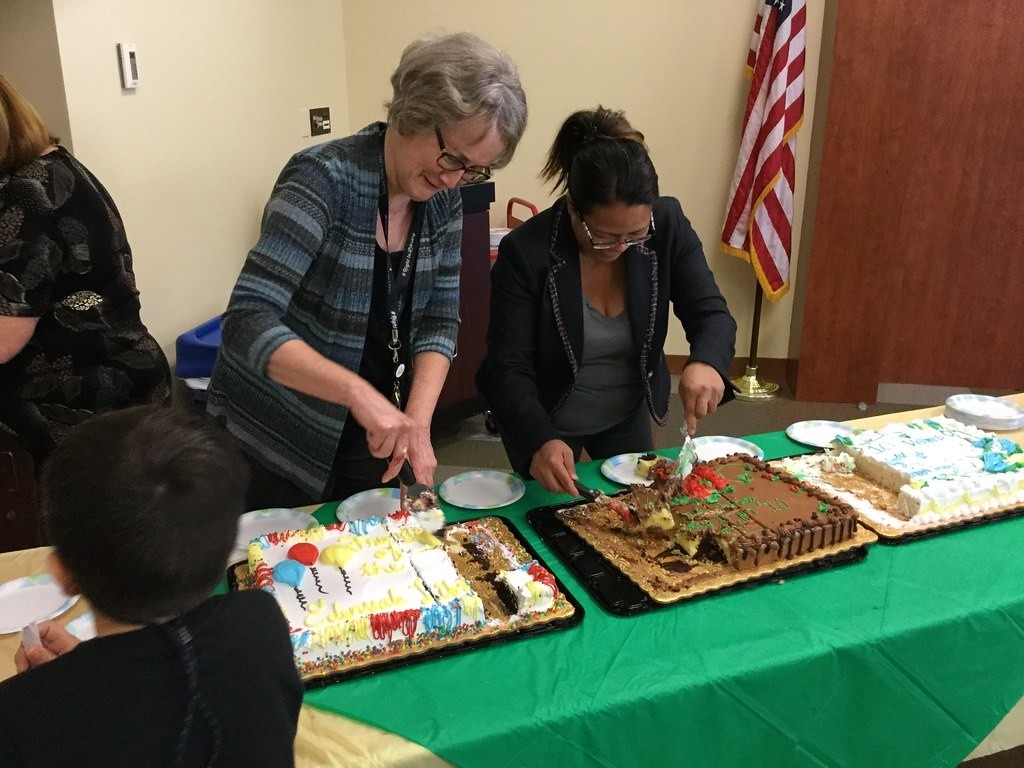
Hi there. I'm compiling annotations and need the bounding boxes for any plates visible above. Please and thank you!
[0,573,82,635]
[232,508,318,551]
[944,394,1023,431]
[437,470,526,510]
[336,487,402,522]
[600,453,676,485]
[691,435,764,461]
[785,419,859,448]
[63,610,97,641]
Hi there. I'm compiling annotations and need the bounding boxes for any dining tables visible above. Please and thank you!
[0,391,1023,768]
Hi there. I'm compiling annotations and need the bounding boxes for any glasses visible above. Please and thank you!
[574,206,656,250]
[435,126,491,185]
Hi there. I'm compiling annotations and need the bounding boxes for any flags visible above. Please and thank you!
[718,1,808,303]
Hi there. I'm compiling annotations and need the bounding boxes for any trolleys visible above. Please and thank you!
[489,197,537,265]
[427,179,503,447]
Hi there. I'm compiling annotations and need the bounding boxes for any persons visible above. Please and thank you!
[475,106,738,497]
[202,31,528,513]
[0,71,173,547]
[0,403,304,768]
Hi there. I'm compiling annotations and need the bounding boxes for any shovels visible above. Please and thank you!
[386,447,446,539]
[654,418,699,512]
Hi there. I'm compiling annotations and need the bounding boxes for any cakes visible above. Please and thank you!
[628,450,859,574]
[248,488,558,675]
[817,415,1023,523]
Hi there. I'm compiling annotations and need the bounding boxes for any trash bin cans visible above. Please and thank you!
[174,311,227,429]
